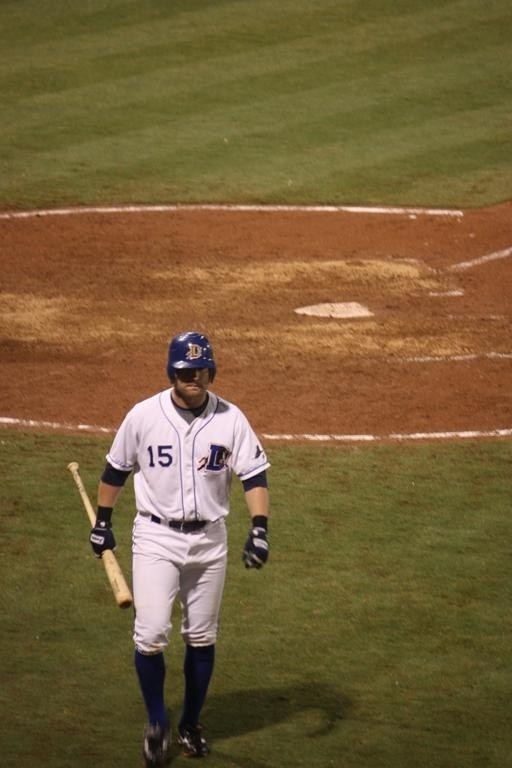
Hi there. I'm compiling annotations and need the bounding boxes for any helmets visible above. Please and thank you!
[167,332,216,377]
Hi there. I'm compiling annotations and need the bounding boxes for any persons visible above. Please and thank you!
[90,332,270,766]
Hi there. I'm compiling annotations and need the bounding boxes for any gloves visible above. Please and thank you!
[89,505,117,558]
[242,515,269,569]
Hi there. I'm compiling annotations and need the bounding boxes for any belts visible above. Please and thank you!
[151,515,207,531]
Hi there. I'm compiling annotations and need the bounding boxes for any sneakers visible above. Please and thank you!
[143,724,172,768]
[176,725,209,759]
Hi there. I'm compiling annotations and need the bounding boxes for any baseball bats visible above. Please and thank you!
[67,460,133,610]
